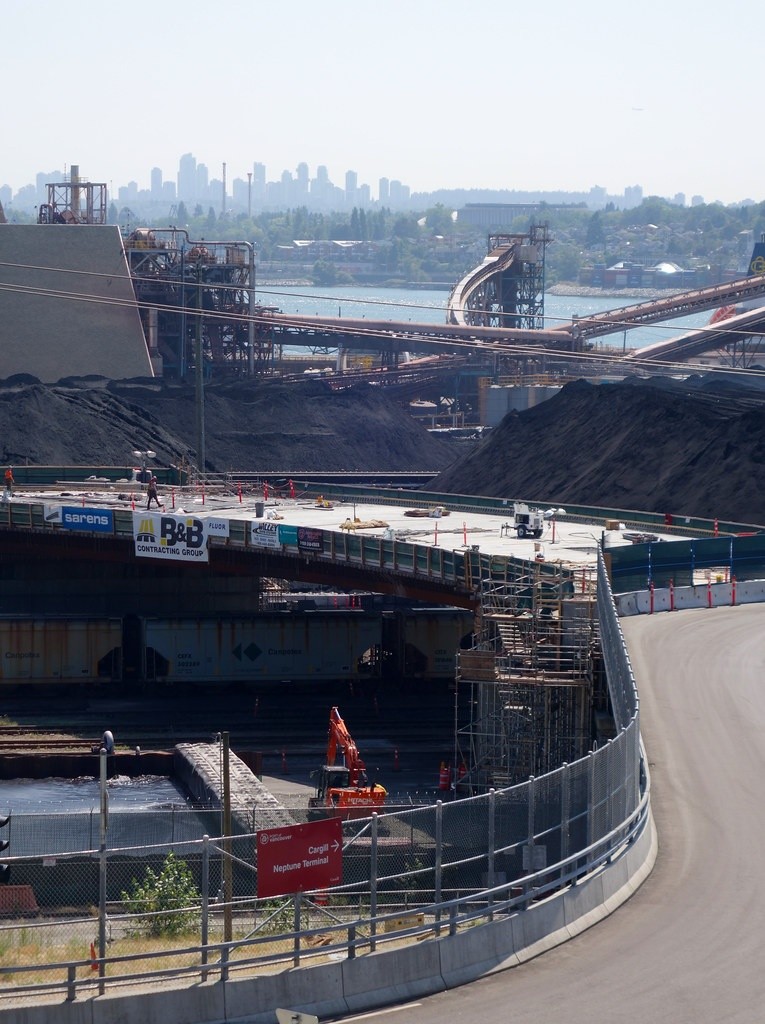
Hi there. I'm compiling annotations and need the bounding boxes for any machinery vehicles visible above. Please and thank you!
[513,502,545,538]
[307,707,390,838]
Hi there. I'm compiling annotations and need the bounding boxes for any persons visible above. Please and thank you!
[147,476,164,510]
[5,465,15,491]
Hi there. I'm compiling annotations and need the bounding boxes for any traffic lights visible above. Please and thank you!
[0,815,11,886]
